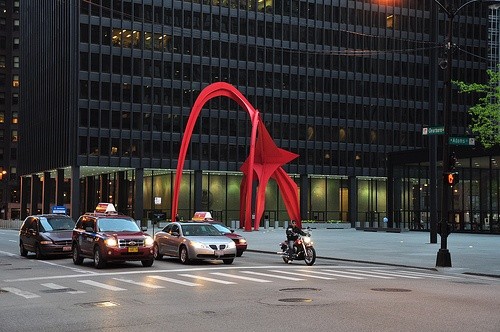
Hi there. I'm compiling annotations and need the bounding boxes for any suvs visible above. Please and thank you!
[72,212,157,270]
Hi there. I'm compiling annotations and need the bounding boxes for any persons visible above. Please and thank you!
[286,220,307,263]
[195,225,202,233]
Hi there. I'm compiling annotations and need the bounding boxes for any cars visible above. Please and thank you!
[154,221,236,264]
[189,212,214,223]
[209,222,247,256]
[19,214,75,259]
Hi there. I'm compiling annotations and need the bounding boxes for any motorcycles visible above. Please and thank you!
[278,226,316,266]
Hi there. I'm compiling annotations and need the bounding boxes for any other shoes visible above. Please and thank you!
[288,260,292,263]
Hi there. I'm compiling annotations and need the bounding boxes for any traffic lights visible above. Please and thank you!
[447,171,460,185]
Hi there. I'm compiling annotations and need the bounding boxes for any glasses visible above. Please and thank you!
[292,223,296,224]
